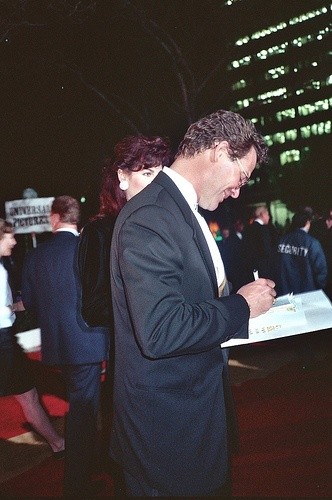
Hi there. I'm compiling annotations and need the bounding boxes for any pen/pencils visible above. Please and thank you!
[253,268,260,283]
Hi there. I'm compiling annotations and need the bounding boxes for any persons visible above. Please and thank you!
[208,201,332,296]
[108,111,277,497]
[78,134,171,497]
[274,212,327,295]
[0,218,66,462]
[20,197,108,497]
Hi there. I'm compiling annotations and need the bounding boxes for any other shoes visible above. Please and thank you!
[48,434,67,460]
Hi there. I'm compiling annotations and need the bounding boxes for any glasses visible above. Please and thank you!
[232,147,251,188]
